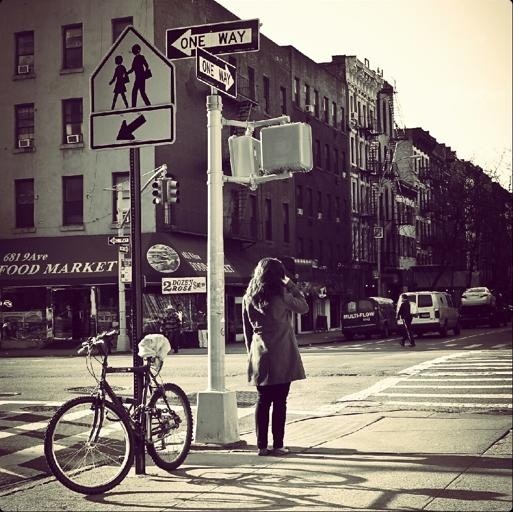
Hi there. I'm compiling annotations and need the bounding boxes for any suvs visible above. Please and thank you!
[394,291,462,339]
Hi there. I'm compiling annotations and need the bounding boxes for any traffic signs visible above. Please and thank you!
[165,17,261,61]
[194,45,239,100]
[117,244,129,253]
[107,235,130,246]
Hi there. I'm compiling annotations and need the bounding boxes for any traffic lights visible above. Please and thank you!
[163,177,180,206]
[151,177,165,206]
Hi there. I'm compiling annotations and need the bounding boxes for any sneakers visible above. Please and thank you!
[273,447,291,456]
[258,448,271,456]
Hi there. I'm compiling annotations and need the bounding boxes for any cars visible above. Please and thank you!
[459,286,497,310]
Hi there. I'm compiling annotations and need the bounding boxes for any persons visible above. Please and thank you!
[241,257,310,455]
[159,304,181,353]
[395,294,416,347]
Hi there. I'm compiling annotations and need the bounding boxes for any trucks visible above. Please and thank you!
[458,287,507,330]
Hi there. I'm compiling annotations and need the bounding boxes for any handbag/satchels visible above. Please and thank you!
[409,302,418,315]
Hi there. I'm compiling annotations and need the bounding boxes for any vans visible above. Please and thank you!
[339,295,398,341]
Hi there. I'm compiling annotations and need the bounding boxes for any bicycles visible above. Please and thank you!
[43,328,194,497]
